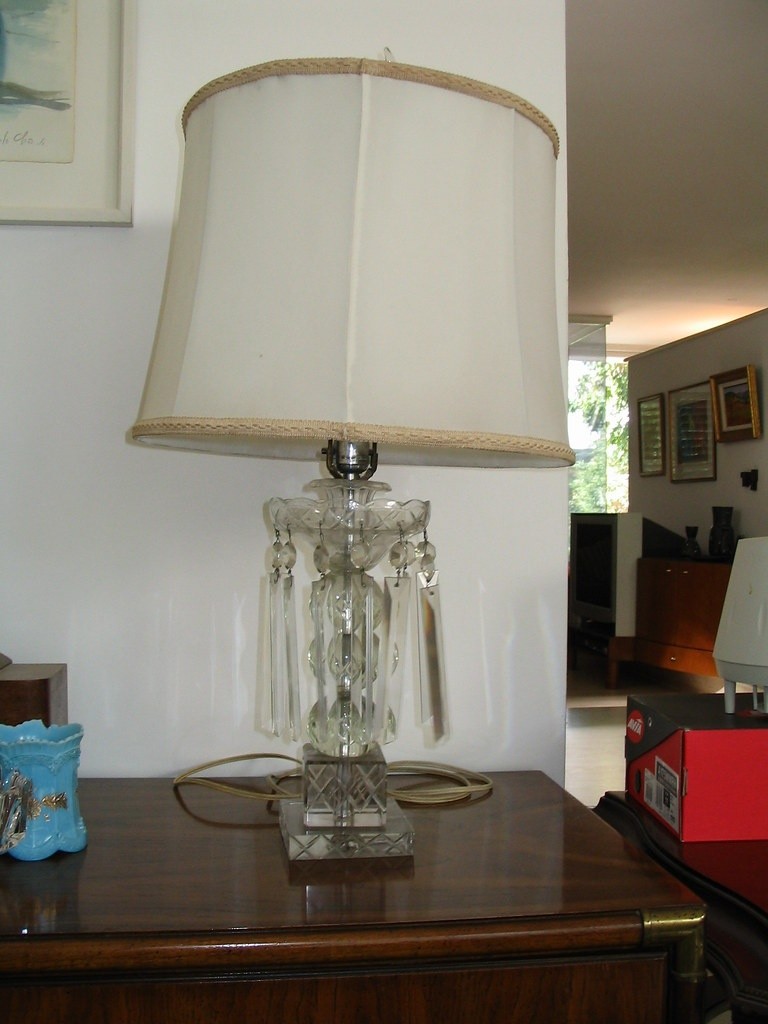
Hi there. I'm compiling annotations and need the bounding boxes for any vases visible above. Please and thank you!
[681,527,701,560]
[708,506,735,561]
[0,719,88,861]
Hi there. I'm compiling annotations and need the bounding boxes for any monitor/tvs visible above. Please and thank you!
[571,513,686,637]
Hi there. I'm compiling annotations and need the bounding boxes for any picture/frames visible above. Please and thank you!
[710,363,760,441]
[636,393,667,477]
[0,0,137,229]
[667,380,716,484]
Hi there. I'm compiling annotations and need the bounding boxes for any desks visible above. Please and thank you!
[592,790,768,1024]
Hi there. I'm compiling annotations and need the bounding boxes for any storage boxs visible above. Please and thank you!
[624,692,768,843]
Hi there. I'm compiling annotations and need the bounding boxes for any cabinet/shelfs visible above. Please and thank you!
[0,769,708,1024]
[636,558,733,677]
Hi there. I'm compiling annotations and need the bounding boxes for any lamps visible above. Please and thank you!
[128,56,577,862]
